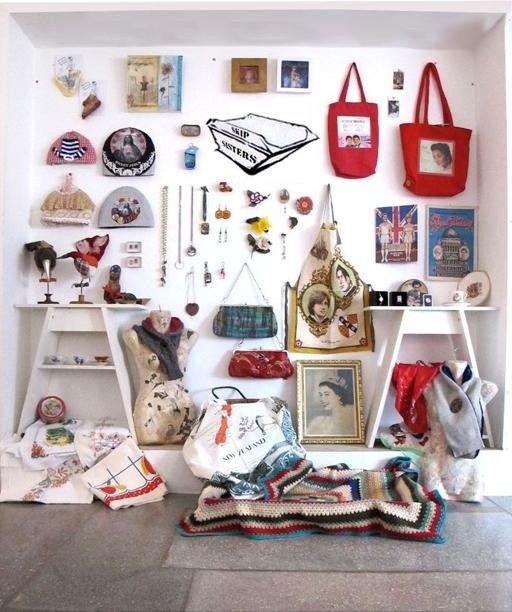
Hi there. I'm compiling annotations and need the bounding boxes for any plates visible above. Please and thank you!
[457,271,490,305]
[400,279,428,306]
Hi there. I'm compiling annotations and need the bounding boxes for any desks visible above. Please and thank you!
[15,303,146,444]
[362,306,498,449]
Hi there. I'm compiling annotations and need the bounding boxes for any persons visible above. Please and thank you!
[306,376,357,437]
[138,74,151,105]
[377,212,393,262]
[290,65,303,87]
[434,248,442,260]
[408,282,425,303]
[430,142,455,173]
[121,134,143,164]
[308,290,331,324]
[121,310,199,445]
[336,264,358,299]
[400,214,415,261]
[345,135,365,147]
[422,360,499,503]
[460,248,468,260]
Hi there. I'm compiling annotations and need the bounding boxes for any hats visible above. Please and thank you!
[98,186,154,228]
[47,131,97,164]
[41,174,96,225]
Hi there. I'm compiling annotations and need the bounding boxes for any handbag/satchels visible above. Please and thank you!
[399,122,472,197]
[328,102,378,179]
[182,398,298,482]
[229,351,295,379]
[213,306,279,337]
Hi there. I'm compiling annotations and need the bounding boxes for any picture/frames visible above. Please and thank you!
[297,359,363,444]
[277,57,313,95]
[425,205,479,282]
